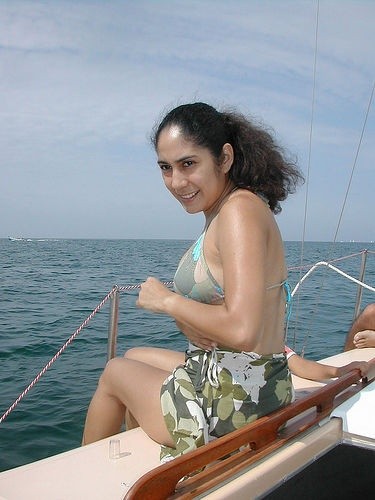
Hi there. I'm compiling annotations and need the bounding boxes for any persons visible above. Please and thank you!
[283,342,370,384]
[79,102,304,455]
[341,301,375,353]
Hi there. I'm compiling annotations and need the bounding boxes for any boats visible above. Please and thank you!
[8,236,32,242]
